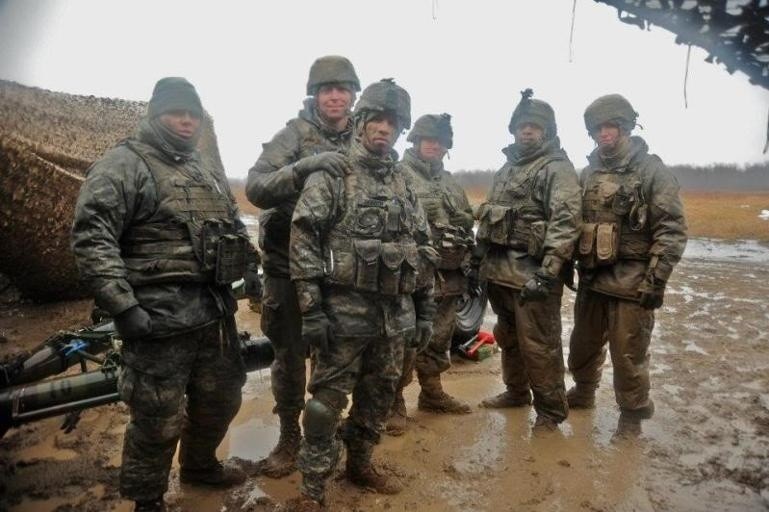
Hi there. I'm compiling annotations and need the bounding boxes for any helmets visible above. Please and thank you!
[406,114,452,150]
[352,79,411,130]
[509,99,556,139]
[307,55,360,95]
[583,95,637,132]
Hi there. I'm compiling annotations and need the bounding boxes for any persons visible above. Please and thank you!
[383,114,474,439]
[460,86,585,439]
[71,76,262,511]
[288,75,444,509]
[564,94,688,449]
[244,56,362,480]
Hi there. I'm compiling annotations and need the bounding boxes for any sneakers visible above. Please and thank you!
[613,410,641,440]
[479,389,532,407]
[531,414,557,440]
[563,386,594,409]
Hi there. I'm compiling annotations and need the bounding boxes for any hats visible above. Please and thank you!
[148,77,203,125]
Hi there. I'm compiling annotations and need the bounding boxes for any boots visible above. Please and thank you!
[180,449,245,486]
[266,406,302,476]
[418,371,471,414]
[385,384,408,432]
[346,440,404,494]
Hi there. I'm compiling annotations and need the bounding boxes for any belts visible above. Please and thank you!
[466,265,483,299]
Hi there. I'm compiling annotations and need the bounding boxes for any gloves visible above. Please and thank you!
[563,260,578,292]
[294,152,355,179]
[412,319,433,354]
[302,309,335,354]
[449,210,473,230]
[636,280,664,311]
[114,306,152,337]
[518,276,551,308]
[243,263,263,298]
